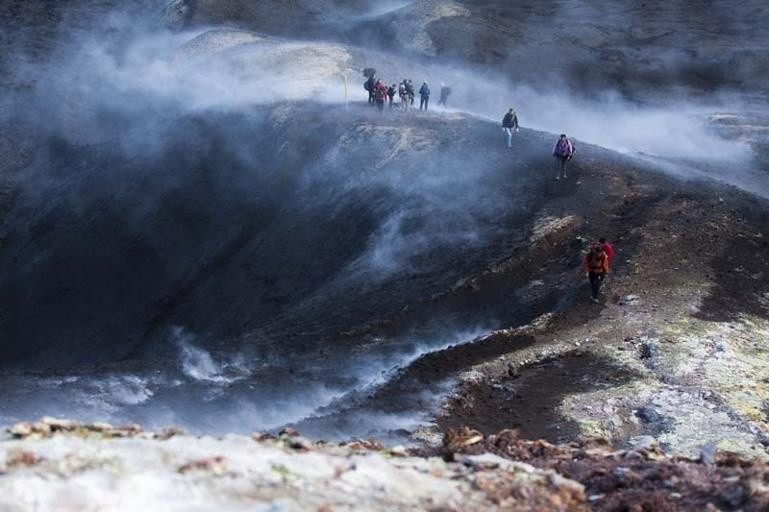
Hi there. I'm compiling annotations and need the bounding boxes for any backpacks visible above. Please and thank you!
[566,140,576,159]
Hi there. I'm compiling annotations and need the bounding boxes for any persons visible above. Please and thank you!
[419,81,430,112]
[583,238,613,304]
[437,82,450,108]
[366,74,414,112]
[502,108,519,150]
[552,134,573,180]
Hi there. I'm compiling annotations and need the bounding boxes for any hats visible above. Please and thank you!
[593,240,600,245]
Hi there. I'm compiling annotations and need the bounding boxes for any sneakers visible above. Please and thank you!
[590,295,600,304]
